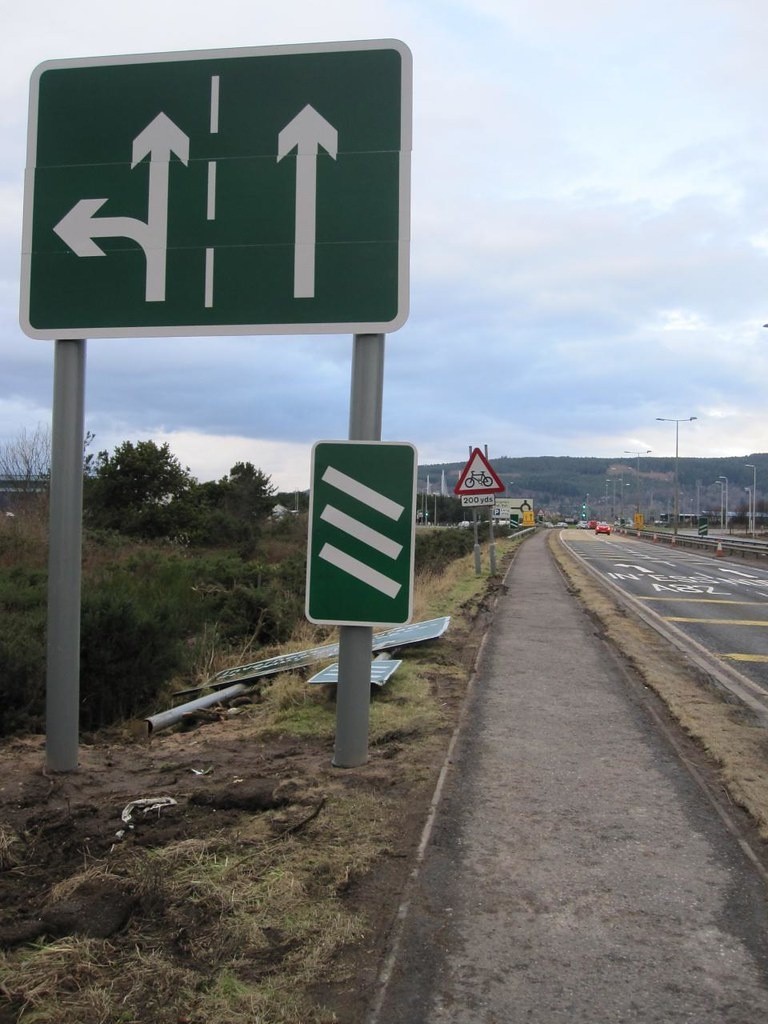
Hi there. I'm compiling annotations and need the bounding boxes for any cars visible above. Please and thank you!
[575,520,600,530]
[543,522,568,529]
[595,523,610,536]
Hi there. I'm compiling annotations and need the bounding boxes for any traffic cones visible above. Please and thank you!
[715,541,724,557]
[653,533,658,543]
[611,527,628,537]
[670,536,678,547]
[637,532,641,539]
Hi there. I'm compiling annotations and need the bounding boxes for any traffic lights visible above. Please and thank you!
[582,503,585,509]
[582,513,586,521]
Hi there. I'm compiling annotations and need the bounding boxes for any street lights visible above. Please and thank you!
[718,475,728,529]
[715,481,724,529]
[656,417,699,534]
[745,465,755,540]
[744,487,751,533]
[605,479,622,520]
[623,450,652,514]
